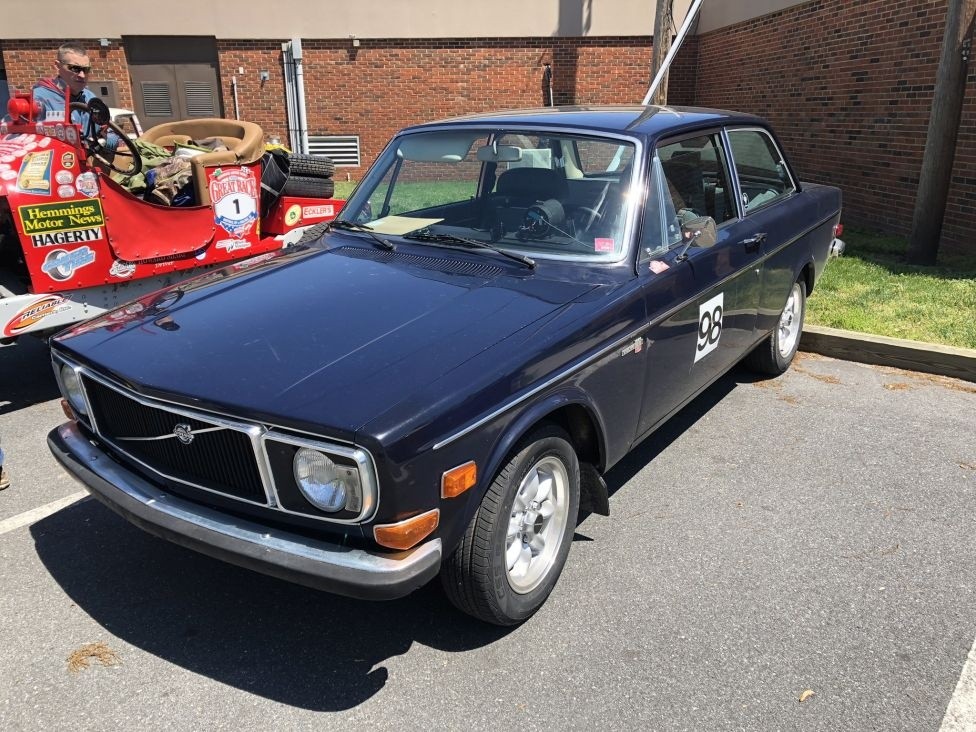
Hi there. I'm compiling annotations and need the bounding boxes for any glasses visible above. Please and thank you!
[58,60,92,75]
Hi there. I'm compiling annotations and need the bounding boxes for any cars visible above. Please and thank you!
[44,102,843,629]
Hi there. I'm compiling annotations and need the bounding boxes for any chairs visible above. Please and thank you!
[618,160,708,216]
[206,136,242,148]
[154,135,192,145]
[480,166,568,231]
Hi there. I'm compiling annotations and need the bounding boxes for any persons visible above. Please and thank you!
[610,162,707,254]
[31,43,119,175]
